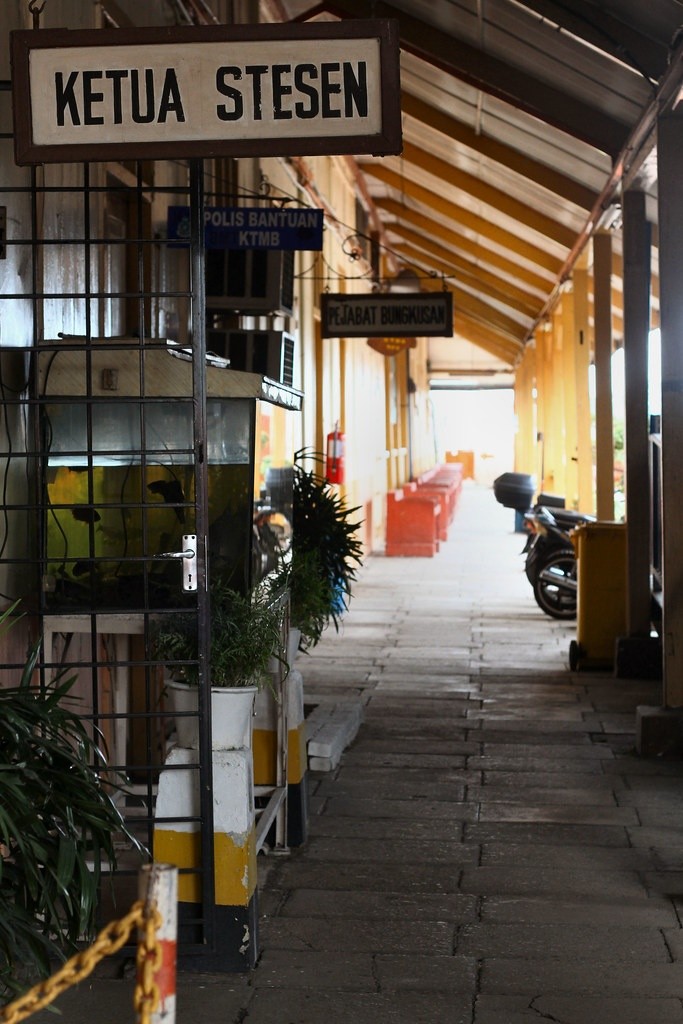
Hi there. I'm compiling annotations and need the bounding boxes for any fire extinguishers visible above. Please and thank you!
[327,420,345,485]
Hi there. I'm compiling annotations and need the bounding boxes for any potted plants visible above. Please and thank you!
[262,445,367,673]
[143,585,286,751]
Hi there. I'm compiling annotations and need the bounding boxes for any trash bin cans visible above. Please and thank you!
[567,522,628,671]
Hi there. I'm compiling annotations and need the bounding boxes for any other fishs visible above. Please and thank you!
[72,479,248,577]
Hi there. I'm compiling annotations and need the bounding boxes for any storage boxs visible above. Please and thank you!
[39,339,305,614]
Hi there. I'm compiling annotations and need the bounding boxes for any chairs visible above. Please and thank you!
[388,460,462,557]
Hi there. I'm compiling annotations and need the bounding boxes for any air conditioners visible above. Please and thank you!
[204,244,295,317]
[206,329,296,389]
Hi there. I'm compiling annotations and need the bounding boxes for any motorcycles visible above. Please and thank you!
[495,472,596,620]
